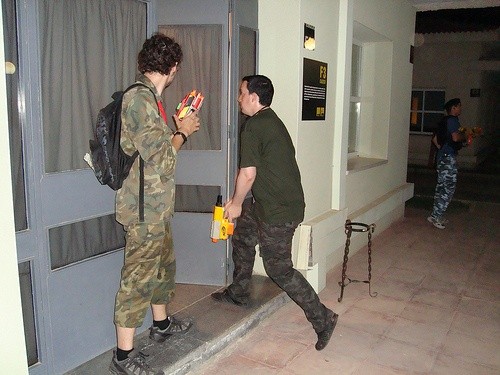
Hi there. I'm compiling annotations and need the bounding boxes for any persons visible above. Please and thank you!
[211,75,339,351]
[427,98,483,230]
[89,32,200,375]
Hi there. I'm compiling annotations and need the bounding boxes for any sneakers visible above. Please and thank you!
[148,315,192,344]
[427,216,446,229]
[108,348,165,375]
[441,218,448,224]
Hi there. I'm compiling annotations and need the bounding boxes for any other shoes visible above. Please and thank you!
[315,312,338,351]
[211,290,247,306]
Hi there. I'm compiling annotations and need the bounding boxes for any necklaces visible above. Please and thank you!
[252,105,269,117]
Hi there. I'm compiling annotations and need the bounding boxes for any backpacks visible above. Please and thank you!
[89,83,160,190]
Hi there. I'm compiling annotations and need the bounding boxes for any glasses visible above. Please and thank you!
[176,63,182,72]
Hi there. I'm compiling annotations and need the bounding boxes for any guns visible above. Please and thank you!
[176,88,205,133]
[210,195,235,243]
[454,126,483,144]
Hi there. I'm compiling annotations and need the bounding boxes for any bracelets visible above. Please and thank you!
[173,131,187,145]
